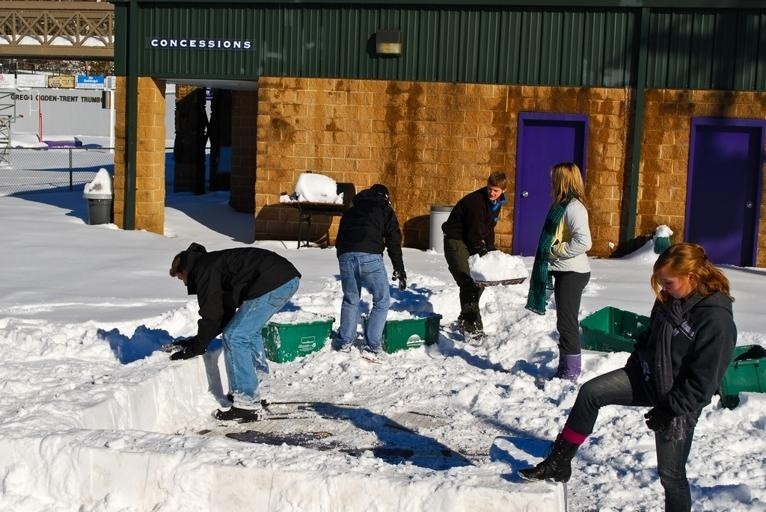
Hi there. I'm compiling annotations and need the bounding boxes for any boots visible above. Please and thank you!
[517,433,580,483]
[535,353,581,391]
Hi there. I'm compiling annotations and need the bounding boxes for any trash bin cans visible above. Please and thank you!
[428,205,458,254]
[83,183,114,225]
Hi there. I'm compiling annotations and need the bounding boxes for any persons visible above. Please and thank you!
[441,171,508,339]
[517,243,737,512]
[169,242,302,419]
[336,184,407,358]
[540,163,594,389]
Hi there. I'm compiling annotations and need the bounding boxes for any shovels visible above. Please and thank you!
[477,278,527,287]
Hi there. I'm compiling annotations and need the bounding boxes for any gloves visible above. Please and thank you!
[392,270,407,291]
[644,401,676,435]
[170,336,205,360]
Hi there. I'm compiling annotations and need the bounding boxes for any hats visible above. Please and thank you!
[370,184,391,205]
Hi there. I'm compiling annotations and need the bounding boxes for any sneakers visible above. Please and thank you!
[363,347,390,363]
[336,342,351,353]
[214,394,270,423]
